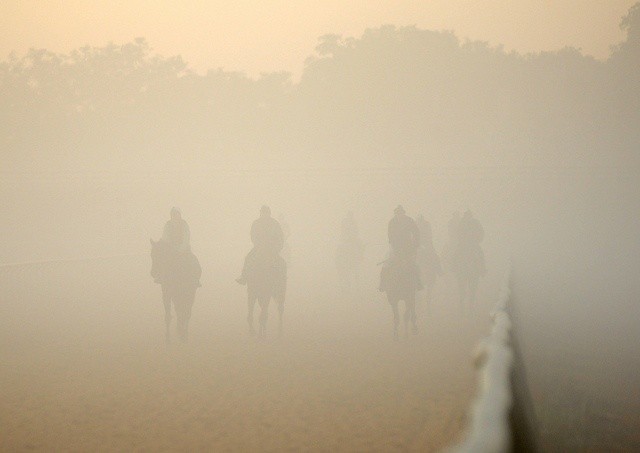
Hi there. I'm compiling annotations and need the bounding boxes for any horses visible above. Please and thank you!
[237,242,286,337]
[379,242,419,342]
[149,237,200,342]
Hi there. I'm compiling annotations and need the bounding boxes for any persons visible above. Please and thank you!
[236,207,284,284]
[162,208,202,287]
[379,205,421,294]
